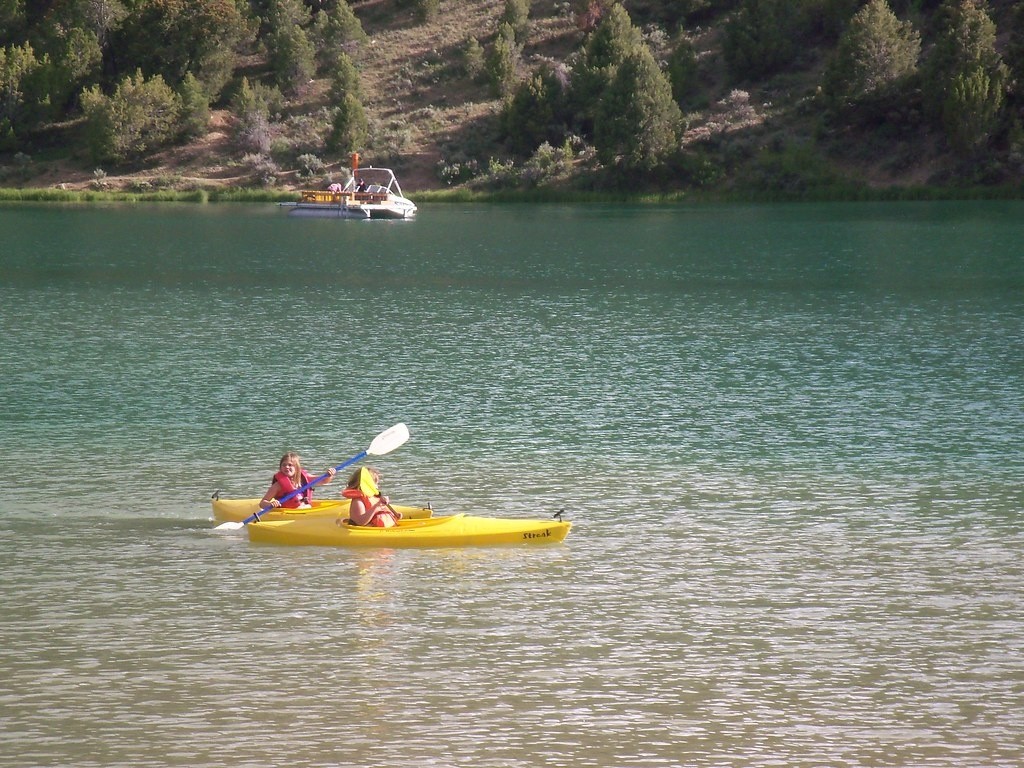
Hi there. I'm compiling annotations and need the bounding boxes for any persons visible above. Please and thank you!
[355,179,367,192]
[348,466,402,527]
[259,451,337,510]
[327,182,341,205]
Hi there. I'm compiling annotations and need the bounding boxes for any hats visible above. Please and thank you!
[359,179,363,182]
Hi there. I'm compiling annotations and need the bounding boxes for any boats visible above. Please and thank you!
[288,168,418,220]
[209,489,572,546]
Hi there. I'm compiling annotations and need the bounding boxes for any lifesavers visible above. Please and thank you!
[305,194,316,201]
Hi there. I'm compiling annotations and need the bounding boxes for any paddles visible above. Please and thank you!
[359,465,400,518]
[214,422,411,531]
